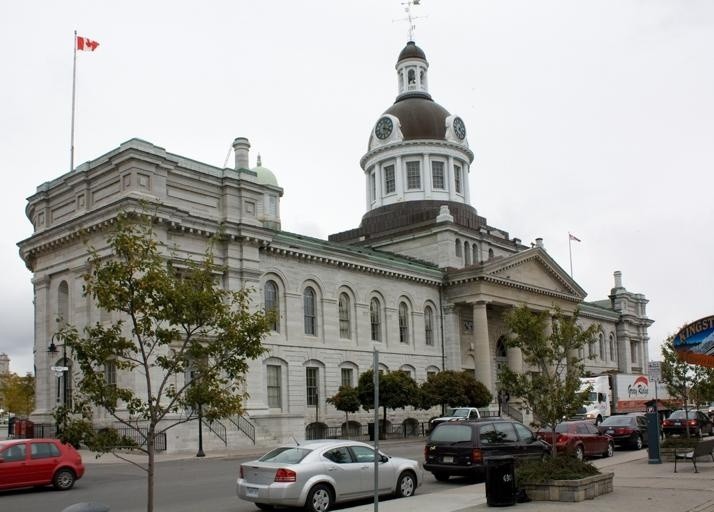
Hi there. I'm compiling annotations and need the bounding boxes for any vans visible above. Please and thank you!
[421,416,554,480]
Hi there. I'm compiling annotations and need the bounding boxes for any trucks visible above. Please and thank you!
[561,371,684,422]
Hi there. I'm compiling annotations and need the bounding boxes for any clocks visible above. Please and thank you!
[375,116,394,140]
[452,116,467,141]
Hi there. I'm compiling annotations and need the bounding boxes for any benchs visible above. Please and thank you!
[673,439,714,476]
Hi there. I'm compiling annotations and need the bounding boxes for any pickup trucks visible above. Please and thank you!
[430,406,481,430]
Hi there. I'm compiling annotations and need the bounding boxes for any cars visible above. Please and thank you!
[661,409,714,439]
[531,420,615,463]
[235,435,425,512]
[596,415,664,450]
[0,437,84,492]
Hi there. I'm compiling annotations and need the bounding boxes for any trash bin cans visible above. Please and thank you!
[8,417,33,439]
[367,422,383,441]
[483,454,517,507]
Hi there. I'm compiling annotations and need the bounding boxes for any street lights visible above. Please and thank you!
[46,332,68,428]
[191,370,206,458]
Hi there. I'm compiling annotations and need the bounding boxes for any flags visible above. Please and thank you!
[76,36,99,52]
[569,234,580,242]
